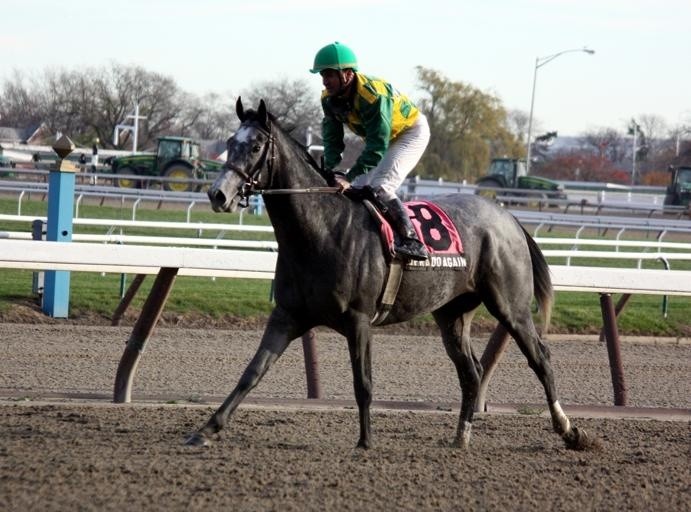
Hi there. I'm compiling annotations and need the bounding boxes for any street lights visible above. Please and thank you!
[524,44,598,179]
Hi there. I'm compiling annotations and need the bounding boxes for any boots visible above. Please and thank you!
[382,197,428,260]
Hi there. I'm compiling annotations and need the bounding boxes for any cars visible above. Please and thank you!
[0,146,14,179]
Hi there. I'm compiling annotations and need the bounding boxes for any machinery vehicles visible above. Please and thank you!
[112,137,230,194]
[474,157,569,211]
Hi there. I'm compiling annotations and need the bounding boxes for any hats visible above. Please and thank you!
[308,39,358,75]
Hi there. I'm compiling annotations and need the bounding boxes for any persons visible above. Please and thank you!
[308,41,433,261]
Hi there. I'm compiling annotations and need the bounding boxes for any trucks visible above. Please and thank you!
[664,164,691,218]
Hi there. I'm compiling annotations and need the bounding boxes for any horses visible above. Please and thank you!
[186,95,589,452]
